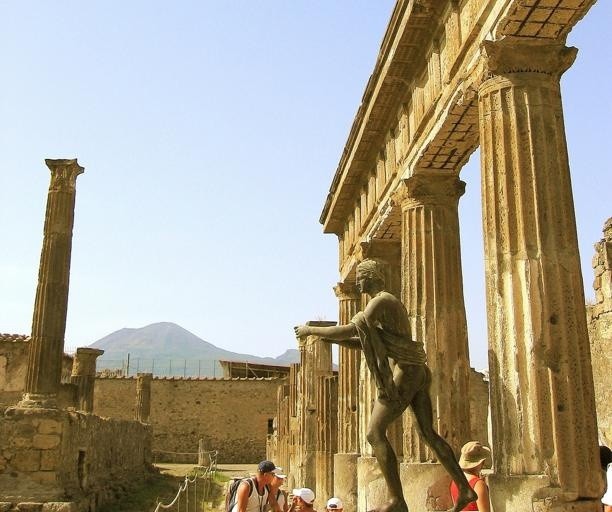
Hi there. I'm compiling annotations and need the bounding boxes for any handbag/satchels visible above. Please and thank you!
[226,478,253,512]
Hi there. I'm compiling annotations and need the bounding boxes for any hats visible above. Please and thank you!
[292,488,315,505]
[257,461,282,474]
[327,498,343,510]
[272,467,287,479]
[459,441,491,470]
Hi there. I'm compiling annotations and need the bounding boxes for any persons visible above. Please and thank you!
[449,441,490,512]
[294,260,478,512]
[601,446,612,512]
[232,461,343,512]
